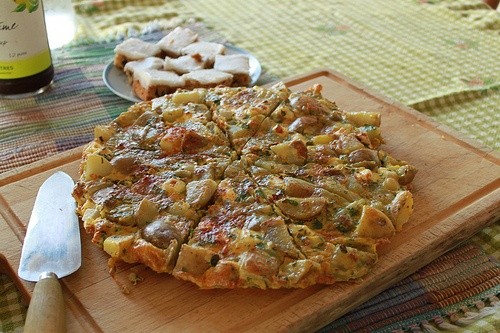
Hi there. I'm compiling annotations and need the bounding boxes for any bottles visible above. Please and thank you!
[0,0,53,98]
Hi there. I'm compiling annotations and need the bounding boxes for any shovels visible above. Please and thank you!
[17,170,82,333]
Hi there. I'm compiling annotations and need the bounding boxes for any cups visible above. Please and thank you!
[43,1,77,49]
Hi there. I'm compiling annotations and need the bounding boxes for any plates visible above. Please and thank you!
[103,38,262,104]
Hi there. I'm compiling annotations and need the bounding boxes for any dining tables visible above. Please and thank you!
[0,0,499,333]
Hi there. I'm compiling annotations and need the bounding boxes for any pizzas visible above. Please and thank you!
[70,84,417,291]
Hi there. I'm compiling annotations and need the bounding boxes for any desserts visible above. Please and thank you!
[112,25,252,101]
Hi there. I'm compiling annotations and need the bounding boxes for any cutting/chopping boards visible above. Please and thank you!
[3,67,499,332]
